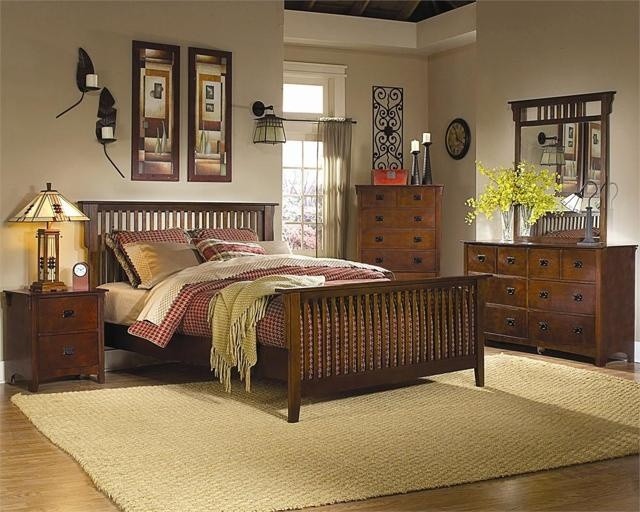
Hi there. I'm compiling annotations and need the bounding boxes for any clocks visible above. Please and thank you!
[445,118,471,161]
[72,262,90,292]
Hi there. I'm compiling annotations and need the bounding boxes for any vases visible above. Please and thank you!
[500,204,515,243]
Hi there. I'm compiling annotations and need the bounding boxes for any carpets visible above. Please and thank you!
[10,352,639,512]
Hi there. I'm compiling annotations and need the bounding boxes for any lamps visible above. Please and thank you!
[560,181,603,246]
[538,132,566,166]
[253,101,286,146]
[596,201,601,209]
[7,182,91,294]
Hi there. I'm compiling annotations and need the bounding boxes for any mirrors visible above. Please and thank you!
[507,90,618,242]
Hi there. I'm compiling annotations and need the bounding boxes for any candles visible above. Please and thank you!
[411,139,419,152]
[423,132,431,144]
[86,74,98,88]
[101,126,114,139]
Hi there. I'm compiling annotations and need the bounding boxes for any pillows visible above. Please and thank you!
[105,226,267,290]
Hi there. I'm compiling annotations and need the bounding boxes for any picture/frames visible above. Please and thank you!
[557,120,584,200]
[187,47,232,182]
[131,40,180,181]
[582,123,602,199]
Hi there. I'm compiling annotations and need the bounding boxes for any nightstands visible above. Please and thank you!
[3,289,110,393]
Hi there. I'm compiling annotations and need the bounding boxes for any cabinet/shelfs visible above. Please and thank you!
[463,241,638,368]
[354,183,445,279]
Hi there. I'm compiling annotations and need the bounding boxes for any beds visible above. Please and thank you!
[76,200,493,423]
